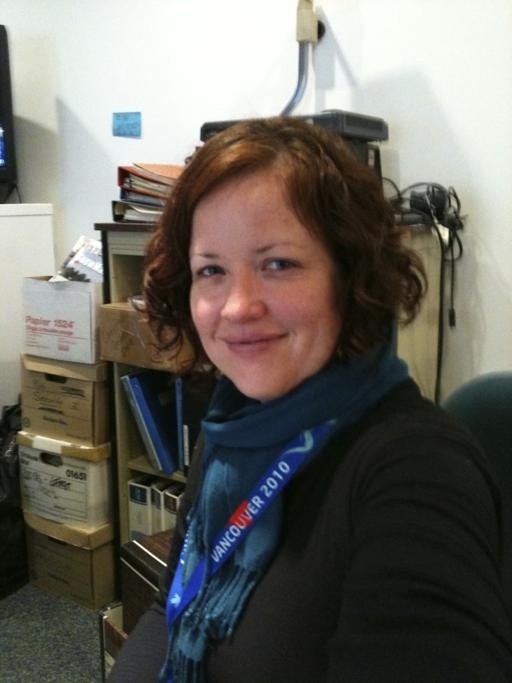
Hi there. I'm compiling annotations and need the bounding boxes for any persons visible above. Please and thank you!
[110,113,511,683]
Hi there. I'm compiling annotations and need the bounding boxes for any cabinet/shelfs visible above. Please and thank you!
[92,221,443,603]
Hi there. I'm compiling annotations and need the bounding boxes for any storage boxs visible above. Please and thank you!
[17,428,115,551]
[15,274,103,367]
[98,298,196,378]
[23,519,117,610]
[16,352,111,447]
